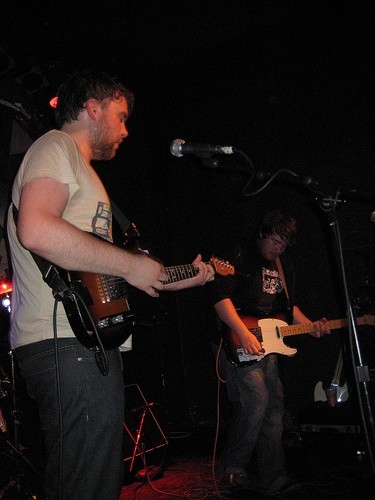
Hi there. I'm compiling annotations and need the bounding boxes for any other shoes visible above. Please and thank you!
[226,470,249,491]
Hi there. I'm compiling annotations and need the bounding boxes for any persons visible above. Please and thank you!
[210,212,333,491]
[4,70,215,500]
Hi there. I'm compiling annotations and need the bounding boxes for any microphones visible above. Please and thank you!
[130,402,158,414]
[170,137,237,160]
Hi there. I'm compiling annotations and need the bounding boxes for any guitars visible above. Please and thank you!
[313,347,350,404]
[230,301,375,365]
[63,229,236,349]
